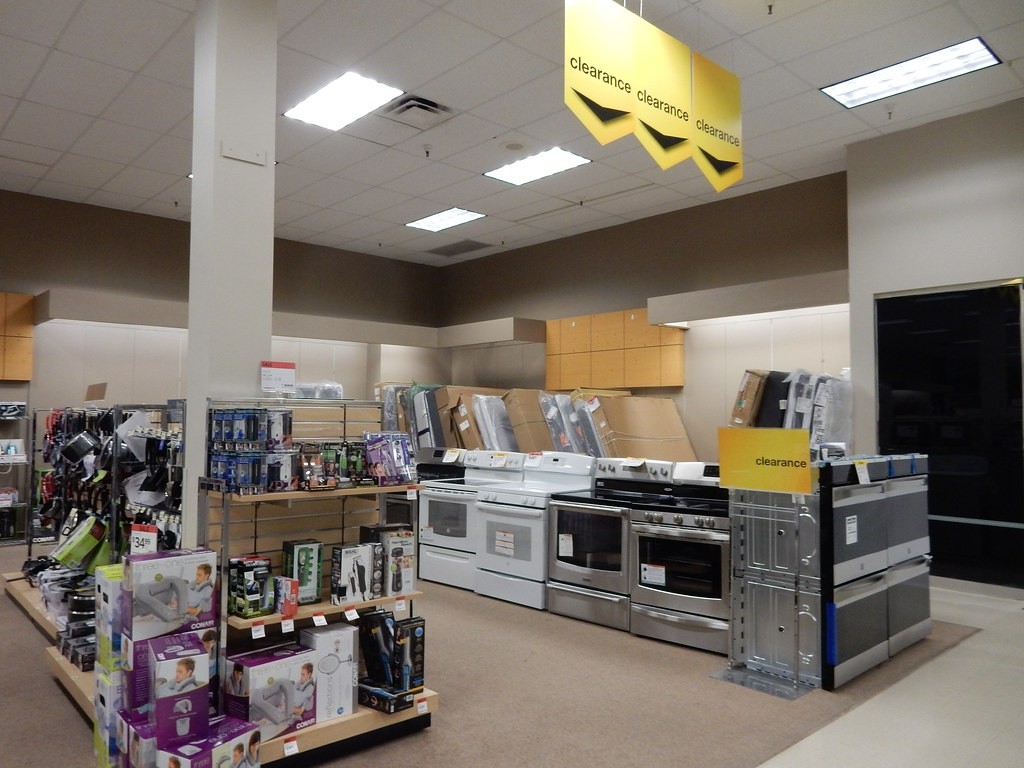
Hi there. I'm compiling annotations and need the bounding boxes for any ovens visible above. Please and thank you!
[737,454,933,690]
[386,446,469,533]
[544,456,674,635]
[416,449,527,591]
[470,449,596,610]
[629,461,731,658]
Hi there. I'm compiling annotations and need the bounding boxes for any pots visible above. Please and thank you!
[23,406,146,627]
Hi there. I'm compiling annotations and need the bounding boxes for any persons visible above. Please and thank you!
[127,731,142,766]
[214,744,247,768]
[154,659,196,697]
[237,730,262,768]
[116,638,134,675]
[168,756,181,768]
[249,660,317,728]
[135,564,215,625]
[199,629,217,676]
[226,662,247,700]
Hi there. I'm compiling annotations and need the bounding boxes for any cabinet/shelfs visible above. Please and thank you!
[3,399,439,768]
